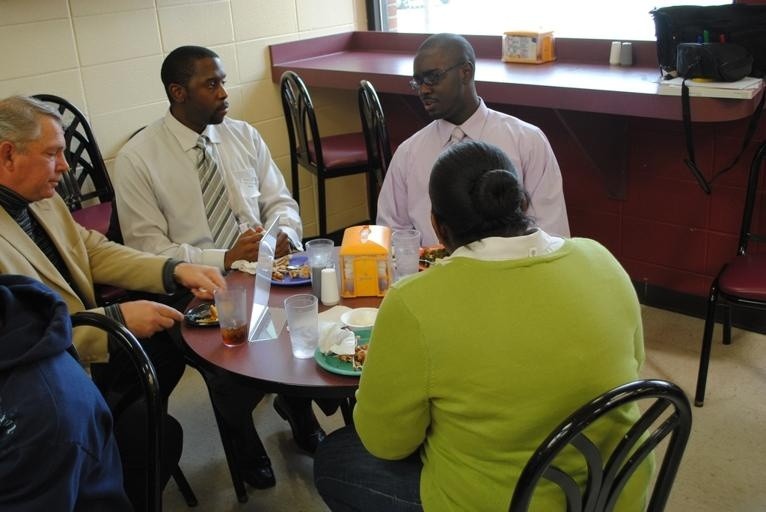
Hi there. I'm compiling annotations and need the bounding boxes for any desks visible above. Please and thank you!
[268,31,765,122]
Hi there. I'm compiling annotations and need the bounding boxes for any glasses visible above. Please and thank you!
[408,60,466,91]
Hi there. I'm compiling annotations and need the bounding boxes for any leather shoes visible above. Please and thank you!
[273,394,329,458]
[229,426,277,490]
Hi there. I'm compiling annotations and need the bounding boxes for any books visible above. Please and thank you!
[656,75,763,99]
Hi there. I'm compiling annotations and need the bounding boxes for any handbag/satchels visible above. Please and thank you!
[652,4,766,82]
[675,41,754,82]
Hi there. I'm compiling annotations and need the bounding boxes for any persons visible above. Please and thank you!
[114,45,328,489]
[314,140,656,511]
[376,32,571,247]
[1,275,136,511]
[0,94,227,432]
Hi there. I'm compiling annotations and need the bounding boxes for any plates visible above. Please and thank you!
[185,303,219,327]
[314,330,372,376]
[258,255,311,285]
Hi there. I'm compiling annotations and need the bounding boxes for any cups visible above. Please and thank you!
[392,229,421,282]
[305,239,334,297]
[213,286,248,348]
[341,308,379,331]
[284,294,319,359]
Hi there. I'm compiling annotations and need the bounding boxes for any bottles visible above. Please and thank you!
[320,269,340,306]
[610,42,621,64]
[622,43,632,64]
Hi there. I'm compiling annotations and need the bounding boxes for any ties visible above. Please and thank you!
[195,136,243,249]
[450,127,466,146]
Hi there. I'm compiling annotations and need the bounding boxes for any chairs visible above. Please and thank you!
[694,143,766,407]
[357,80,394,222]
[62,312,161,511]
[509,378,692,512]
[279,71,406,238]
[25,94,124,246]
